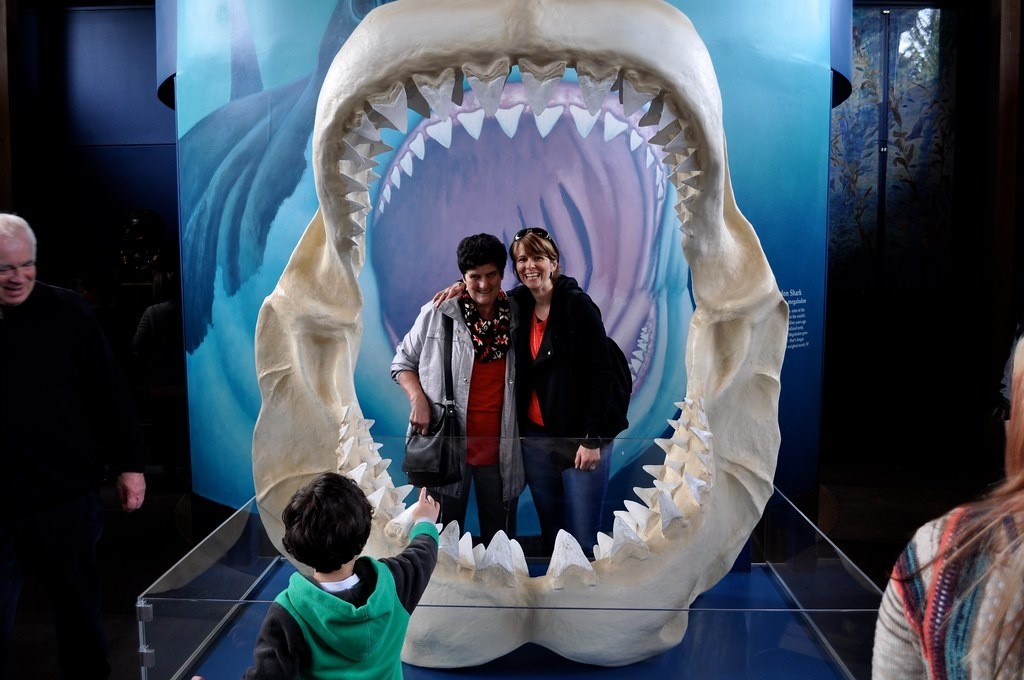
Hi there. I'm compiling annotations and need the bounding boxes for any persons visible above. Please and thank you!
[0,212,147,680]
[193,472,439,680]
[870,332,1024,680]
[391,232,522,552]
[434,228,612,562]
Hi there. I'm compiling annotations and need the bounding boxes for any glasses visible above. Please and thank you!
[514,228,549,241]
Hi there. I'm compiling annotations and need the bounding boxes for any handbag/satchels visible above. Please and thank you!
[402,313,462,488]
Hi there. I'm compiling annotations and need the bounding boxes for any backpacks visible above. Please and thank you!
[582,337,633,449]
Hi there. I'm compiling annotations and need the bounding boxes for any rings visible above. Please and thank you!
[442,291,448,296]
[590,466,595,469]
